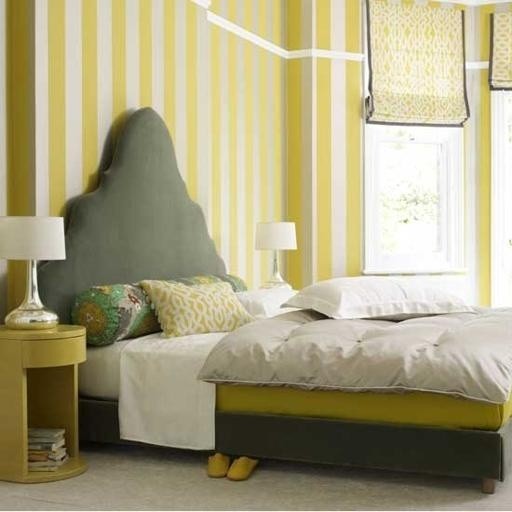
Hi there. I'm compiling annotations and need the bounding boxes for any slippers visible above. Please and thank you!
[208,453,230,477]
[227,456,258,480]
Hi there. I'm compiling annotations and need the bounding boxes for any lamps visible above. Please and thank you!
[255,221,297,289]
[1,215,67,329]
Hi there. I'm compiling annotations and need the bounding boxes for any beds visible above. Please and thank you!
[36,105,511,495]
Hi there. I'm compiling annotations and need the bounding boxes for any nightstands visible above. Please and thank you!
[1,324,89,483]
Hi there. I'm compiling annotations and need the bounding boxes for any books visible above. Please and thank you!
[28,428,72,472]
